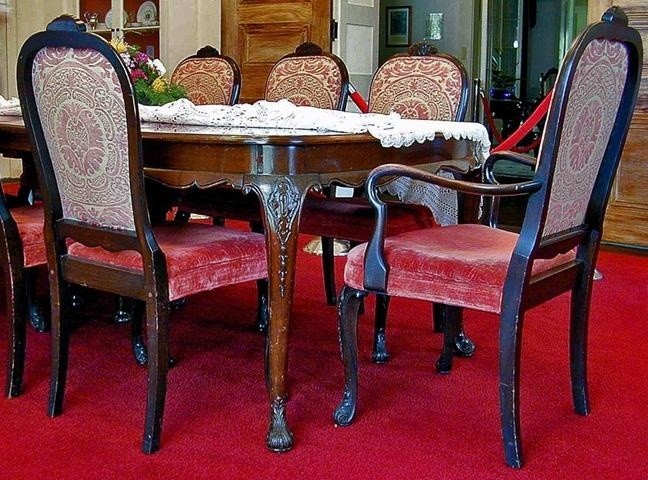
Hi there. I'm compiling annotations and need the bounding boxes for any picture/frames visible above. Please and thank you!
[383,6,413,47]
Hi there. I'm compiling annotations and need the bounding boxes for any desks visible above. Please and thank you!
[485,90,524,138]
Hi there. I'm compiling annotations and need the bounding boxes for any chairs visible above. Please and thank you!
[15,14,271,457]
[333,6,643,469]
[1,185,151,401]
[348,43,476,364]
[539,67,558,100]
[175,42,345,326]
[161,44,240,226]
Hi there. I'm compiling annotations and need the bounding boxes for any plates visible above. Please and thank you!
[104,8,128,28]
[136,1,156,26]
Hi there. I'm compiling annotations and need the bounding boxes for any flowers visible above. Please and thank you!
[106,32,187,108]
